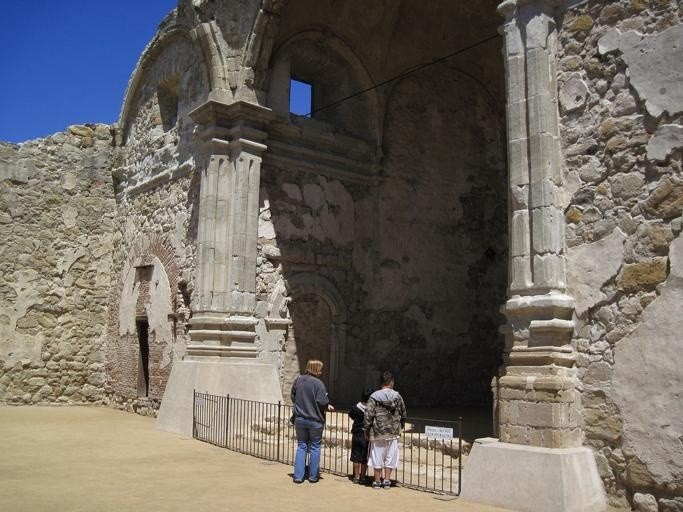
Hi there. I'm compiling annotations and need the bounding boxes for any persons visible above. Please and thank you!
[363,371,407,490]
[348,386,374,484]
[290,359,335,484]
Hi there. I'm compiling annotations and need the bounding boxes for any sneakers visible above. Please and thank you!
[294,475,318,483]
[372,481,382,488]
[353,479,368,484]
[383,480,391,488]
